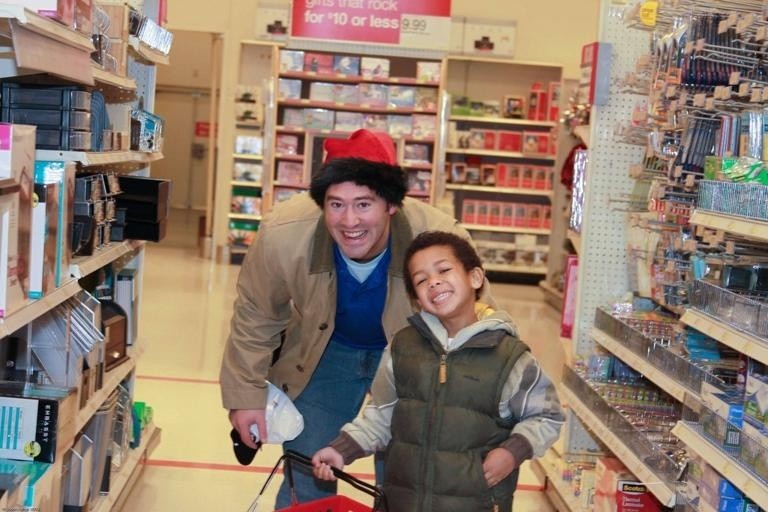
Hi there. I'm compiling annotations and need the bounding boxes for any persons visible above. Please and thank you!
[220,128,501,510]
[311,231,566,512]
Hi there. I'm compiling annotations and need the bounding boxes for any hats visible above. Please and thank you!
[310,130,409,209]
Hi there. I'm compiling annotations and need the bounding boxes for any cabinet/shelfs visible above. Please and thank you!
[2,0,161,511]
[223,39,566,278]
[529,0,768,512]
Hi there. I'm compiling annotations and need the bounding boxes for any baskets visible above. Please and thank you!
[247,450,390,512]
